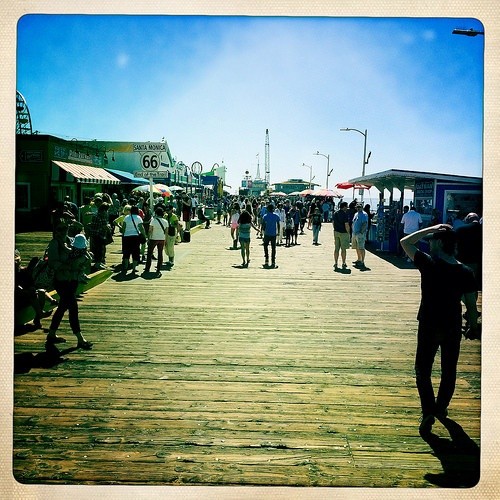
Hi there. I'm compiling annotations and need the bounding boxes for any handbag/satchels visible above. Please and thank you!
[167,225,176,237]
[99,225,113,246]
[138,232,146,245]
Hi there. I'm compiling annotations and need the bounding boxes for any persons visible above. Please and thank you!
[398,224,478,418]
[454,211,482,326]
[16,225,92,354]
[61,189,421,273]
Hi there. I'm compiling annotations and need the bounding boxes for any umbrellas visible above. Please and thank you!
[135,183,184,197]
[337,181,372,200]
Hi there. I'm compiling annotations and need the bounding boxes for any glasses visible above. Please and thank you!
[55,226,68,232]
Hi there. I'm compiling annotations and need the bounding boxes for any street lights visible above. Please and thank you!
[314,150,332,190]
[339,127,371,203]
[301,163,315,191]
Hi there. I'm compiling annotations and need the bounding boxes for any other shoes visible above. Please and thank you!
[419,404,449,434]
[77,254,177,285]
[50,298,60,307]
[33,316,42,329]
[229,231,321,271]
[46,334,93,348]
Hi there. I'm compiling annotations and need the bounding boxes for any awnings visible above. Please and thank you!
[53,160,119,186]
[105,167,155,185]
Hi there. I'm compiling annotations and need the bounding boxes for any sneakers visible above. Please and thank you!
[333,260,365,270]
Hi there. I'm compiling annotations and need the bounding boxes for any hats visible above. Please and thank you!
[354,203,363,208]
[70,234,89,249]
[422,228,452,240]
